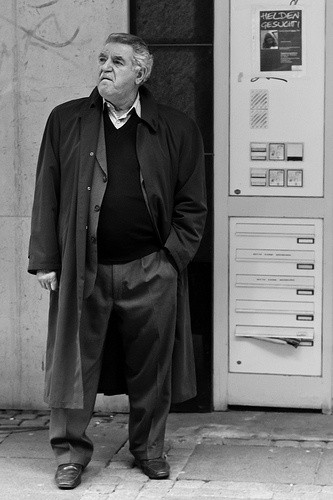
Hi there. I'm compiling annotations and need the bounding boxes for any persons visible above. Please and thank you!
[25,30,210,489]
[262,32,278,49]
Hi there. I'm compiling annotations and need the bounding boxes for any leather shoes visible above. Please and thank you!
[54,463,84,489]
[133,456,170,478]
